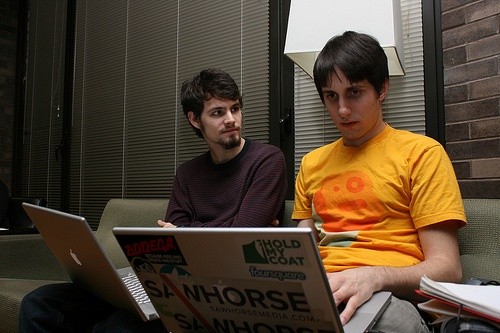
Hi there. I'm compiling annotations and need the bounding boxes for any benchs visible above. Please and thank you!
[0,198,500,333]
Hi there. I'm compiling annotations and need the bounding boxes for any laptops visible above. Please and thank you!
[111,225,393,333]
[21,202,161,324]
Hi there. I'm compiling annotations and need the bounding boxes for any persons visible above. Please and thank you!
[291,31,467,333]
[19,69,288,333]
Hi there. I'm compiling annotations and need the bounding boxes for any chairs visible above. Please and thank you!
[0,198,43,235]
